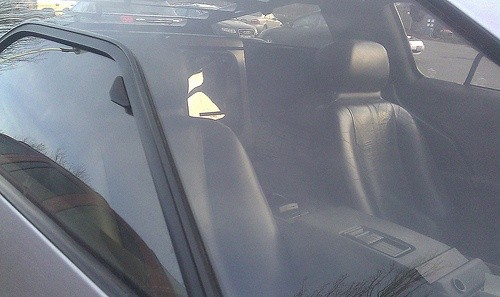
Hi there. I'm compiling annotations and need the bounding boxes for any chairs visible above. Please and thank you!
[72,39,301,297]
[309,37,500,275]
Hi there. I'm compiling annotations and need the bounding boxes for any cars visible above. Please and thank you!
[237,11,282,32]
[63,0,175,26]
[258,10,336,47]
[0,0,500,296]
[176,4,254,38]
